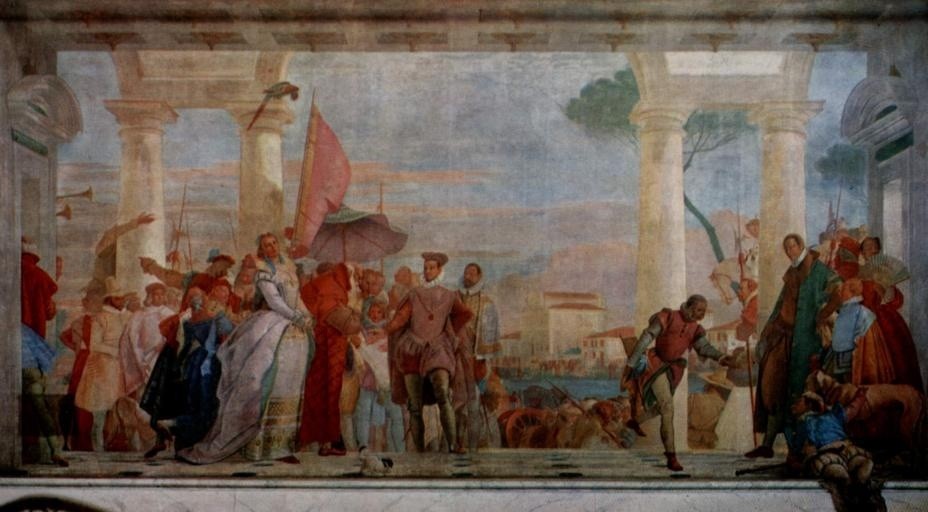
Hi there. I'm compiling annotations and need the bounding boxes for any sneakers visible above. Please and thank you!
[743,443,776,460]
[276,454,301,465]
[317,447,348,458]
[50,454,70,467]
[448,443,467,454]
[143,440,168,459]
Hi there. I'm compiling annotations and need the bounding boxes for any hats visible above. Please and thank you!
[145,282,166,294]
[421,251,450,268]
[99,274,139,305]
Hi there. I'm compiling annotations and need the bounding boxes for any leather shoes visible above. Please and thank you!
[625,418,648,437]
[662,449,684,472]
